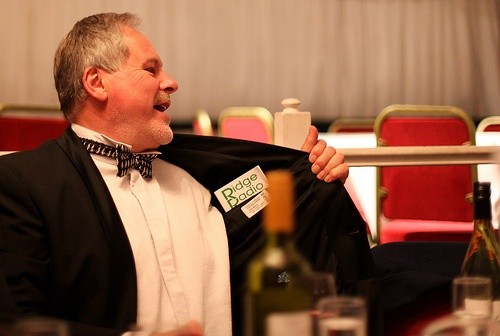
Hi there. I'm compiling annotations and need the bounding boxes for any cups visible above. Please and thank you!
[317,296,368,336]
[310,263,337,336]
[453,277,493,336]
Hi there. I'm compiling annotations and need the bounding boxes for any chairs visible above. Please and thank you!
[0,103,72,157]
[193,102,500,243]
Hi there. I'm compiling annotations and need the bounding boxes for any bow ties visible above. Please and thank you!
[79,138,160,180]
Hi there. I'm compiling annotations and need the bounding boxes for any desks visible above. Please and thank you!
[318,129,500,245]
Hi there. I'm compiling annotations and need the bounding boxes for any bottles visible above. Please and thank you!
[460,181,500,320]
[248,172,313,290]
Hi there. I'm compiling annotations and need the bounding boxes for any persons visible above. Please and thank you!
[0,11,377,336]
